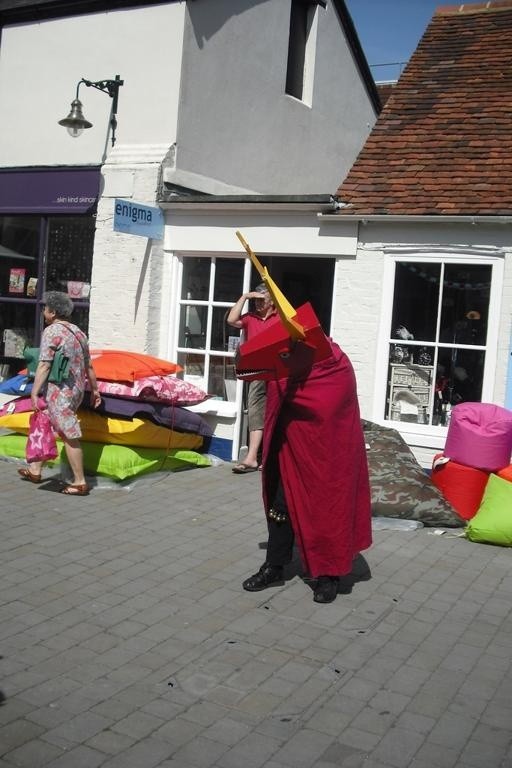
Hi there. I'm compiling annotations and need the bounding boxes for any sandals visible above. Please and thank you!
[18,468,41,483]
[61,485,88,495]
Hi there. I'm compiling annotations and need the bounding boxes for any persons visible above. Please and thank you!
[236,229,373,604]
[225,281,275,473]
[15,289,101,496]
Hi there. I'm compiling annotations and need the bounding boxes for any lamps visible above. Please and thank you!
[59,75,123,146]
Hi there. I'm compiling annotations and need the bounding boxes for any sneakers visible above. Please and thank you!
[314,577,340,603]
[244,562,285,591]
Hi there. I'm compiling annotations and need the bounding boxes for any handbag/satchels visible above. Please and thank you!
[25,409,58,463]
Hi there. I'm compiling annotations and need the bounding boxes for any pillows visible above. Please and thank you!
[0,349,214,481]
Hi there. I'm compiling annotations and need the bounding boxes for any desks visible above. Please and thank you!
[388,363,434,421]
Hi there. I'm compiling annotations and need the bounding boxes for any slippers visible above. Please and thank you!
[232,464,263,472]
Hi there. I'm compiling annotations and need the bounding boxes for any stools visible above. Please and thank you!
[433,401,512,522]
